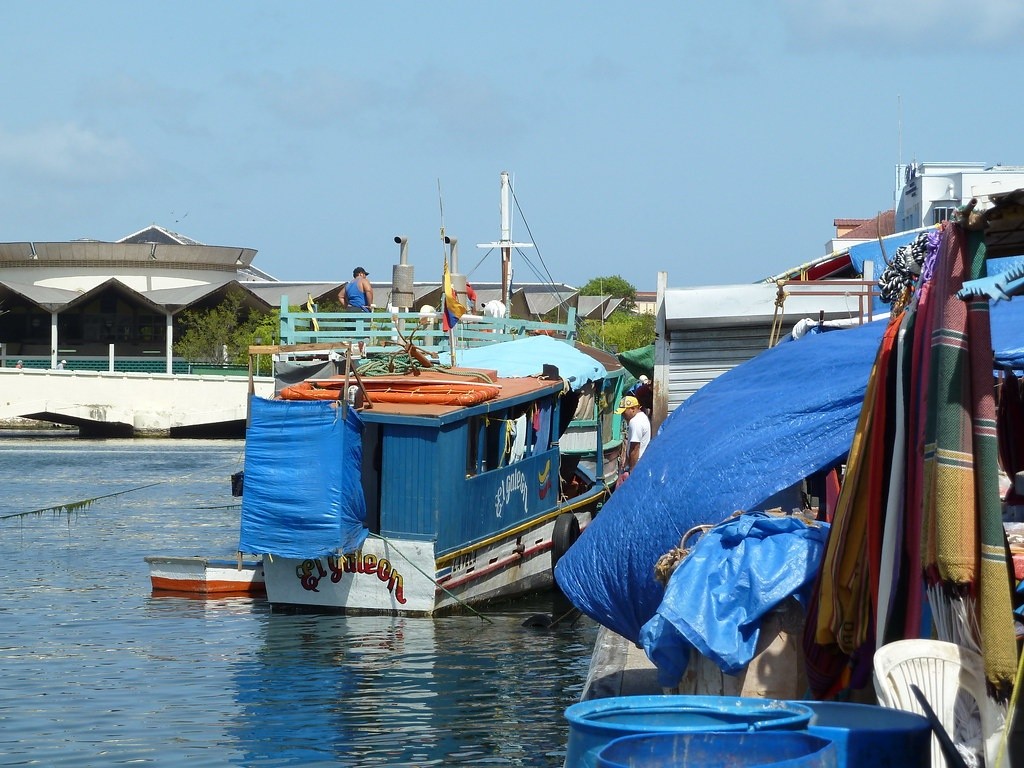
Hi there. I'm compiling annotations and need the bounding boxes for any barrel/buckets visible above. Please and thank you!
[563,693,931,767]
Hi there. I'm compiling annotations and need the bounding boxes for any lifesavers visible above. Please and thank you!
[550,513,583,589]
[521,613,559,629]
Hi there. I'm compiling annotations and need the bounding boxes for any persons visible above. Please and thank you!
[338,267,373,331]
[614,396,650,475]
[637,375,652,414]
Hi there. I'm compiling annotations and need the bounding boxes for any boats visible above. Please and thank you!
[238,171,627,619]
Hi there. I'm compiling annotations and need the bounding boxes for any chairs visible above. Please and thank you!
[872,636,1011,768]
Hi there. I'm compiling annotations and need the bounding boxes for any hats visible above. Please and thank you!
[353,267,369,276]
[614,396,639,414]
